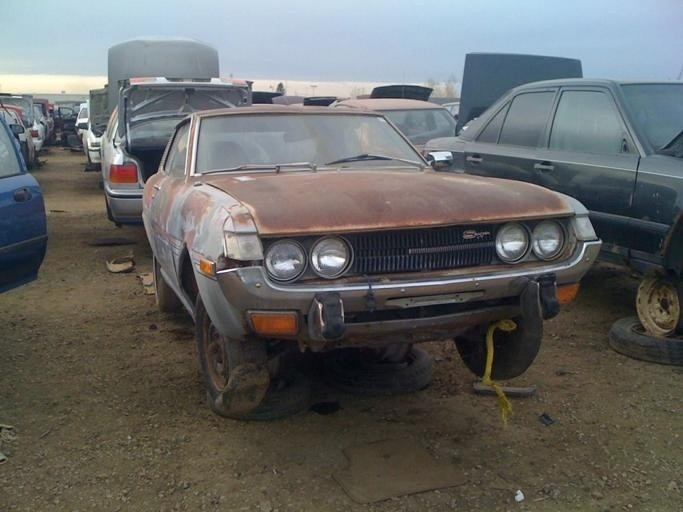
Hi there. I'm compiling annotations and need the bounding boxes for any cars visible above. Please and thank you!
[140,105,604,420]
[421,52,682,341]
[0,115,48,295]
[0,37,221,173]
[254,84,466,178]
[95,80,249,229]
